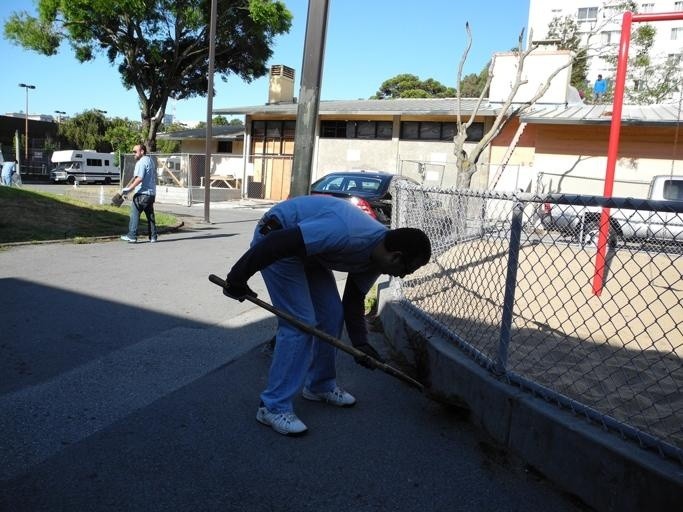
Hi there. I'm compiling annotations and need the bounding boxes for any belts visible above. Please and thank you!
[262,214,269,223]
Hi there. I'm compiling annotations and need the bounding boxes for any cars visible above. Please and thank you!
[287,172,454,241]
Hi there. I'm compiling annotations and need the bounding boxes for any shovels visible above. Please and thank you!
[209,274,472,411]
[112,177,135,207]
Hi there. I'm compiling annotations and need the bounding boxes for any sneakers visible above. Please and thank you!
[120,235,137,243]
[302,386,356,407]
[256,402,308,436]
[151,240,157,243]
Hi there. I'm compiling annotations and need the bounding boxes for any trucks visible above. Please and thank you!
[49,150,122,184]
[157,158,180,181]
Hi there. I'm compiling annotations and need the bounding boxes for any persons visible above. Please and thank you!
[591,73,606,105]
[222,195,430,436]
[0,159,18,186]
[118,144,157,243]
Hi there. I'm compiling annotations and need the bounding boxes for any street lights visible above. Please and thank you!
[127,122,131,137]
[54,110,66,166]
[19,83,36,160]
[98,110,108,136]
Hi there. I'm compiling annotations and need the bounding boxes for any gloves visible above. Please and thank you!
[122,188,129,195]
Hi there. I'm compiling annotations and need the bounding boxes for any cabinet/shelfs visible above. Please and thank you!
[199,176,238,189]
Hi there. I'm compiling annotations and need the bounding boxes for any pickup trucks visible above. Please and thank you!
[543,174,683,250]
